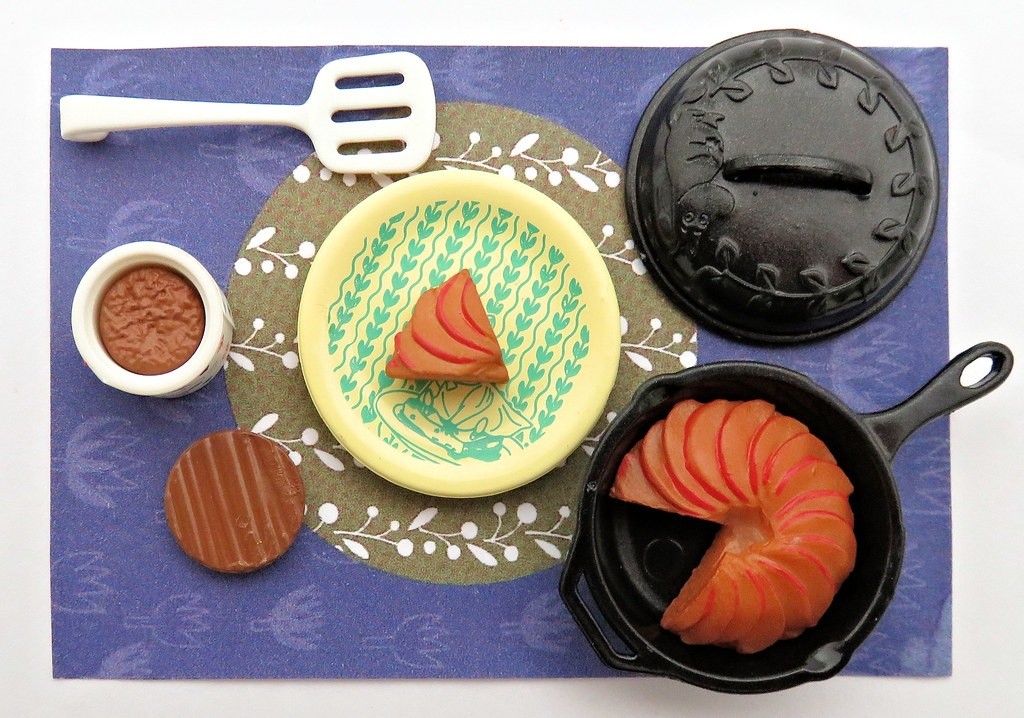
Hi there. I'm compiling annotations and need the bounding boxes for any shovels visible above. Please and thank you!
[60,51,438,176]
